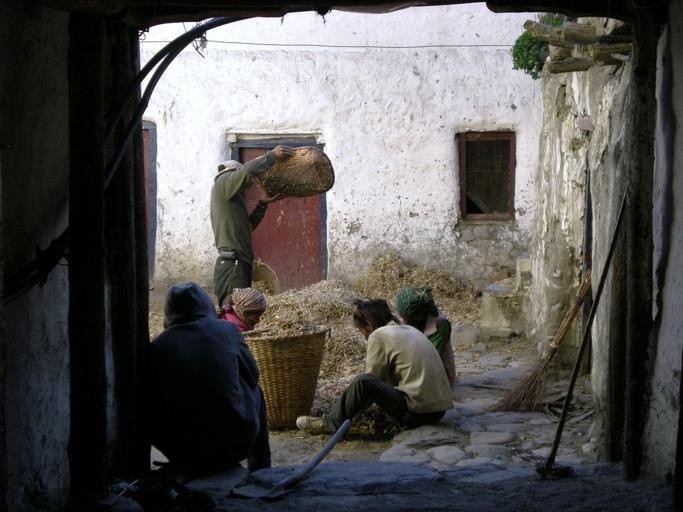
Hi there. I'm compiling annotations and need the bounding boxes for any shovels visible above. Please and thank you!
[230,418,352,499]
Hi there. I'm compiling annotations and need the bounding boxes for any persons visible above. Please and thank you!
[296,295,456,436]
[209,145,296,306]
[215,287,266,334]
[396,284,456,385]
[149,281,272,473]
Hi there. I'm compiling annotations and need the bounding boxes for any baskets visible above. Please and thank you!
[251,258,280,296]
[240,323,329,432]
[252,148,334,197]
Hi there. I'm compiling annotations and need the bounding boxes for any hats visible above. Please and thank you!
[213,159,243,181]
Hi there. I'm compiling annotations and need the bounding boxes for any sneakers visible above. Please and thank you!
[296,412,337,434]
